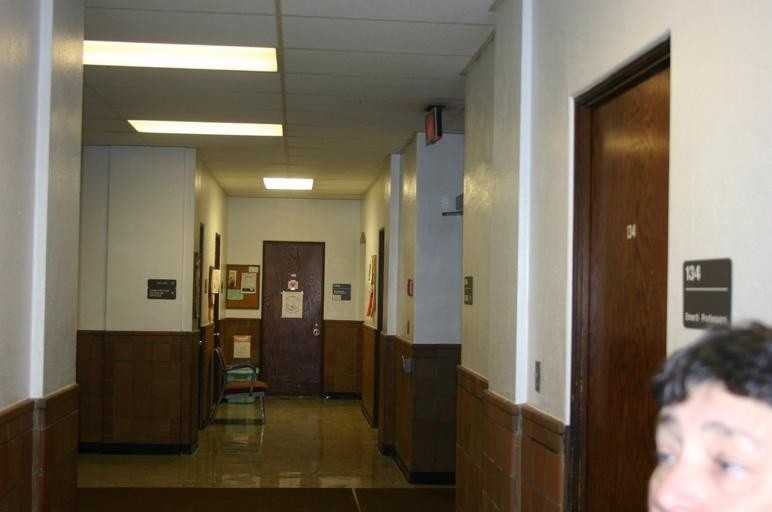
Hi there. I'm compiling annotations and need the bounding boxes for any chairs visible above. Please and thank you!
[201,346,268,429]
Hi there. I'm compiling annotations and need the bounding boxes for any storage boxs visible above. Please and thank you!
[226,365,261,403]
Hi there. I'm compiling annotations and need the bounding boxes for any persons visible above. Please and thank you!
[644,320,771,510]
